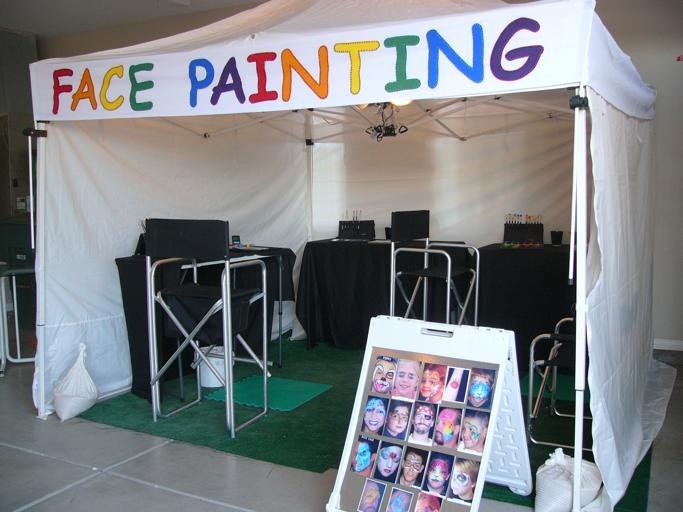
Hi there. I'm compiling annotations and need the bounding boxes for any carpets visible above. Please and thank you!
[76,330,651,512]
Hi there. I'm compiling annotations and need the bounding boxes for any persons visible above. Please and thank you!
[349,355,496,512]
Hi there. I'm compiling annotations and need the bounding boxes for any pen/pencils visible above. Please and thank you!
[352,210,361,221]
[505,213,541,224]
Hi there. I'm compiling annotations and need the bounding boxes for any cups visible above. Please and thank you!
[550,230,562,247]
